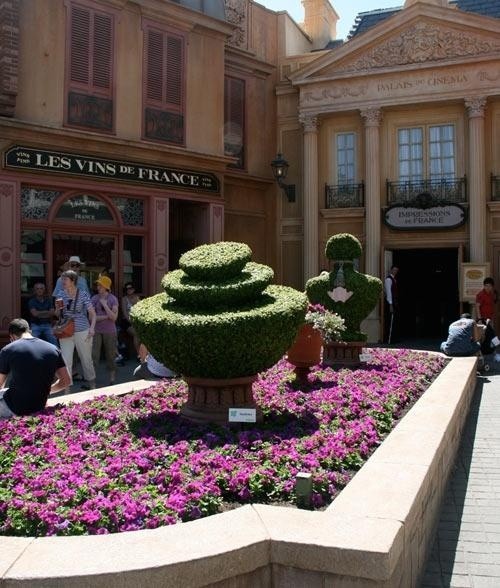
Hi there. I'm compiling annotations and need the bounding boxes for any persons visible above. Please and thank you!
[440,311,482,376]
[1,317,72,418]
[29,254,140,395]
[384,264,400,343]
[475,277,500,327]
[132,343,181,381]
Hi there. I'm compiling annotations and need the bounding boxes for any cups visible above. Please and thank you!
[55,298,63,309]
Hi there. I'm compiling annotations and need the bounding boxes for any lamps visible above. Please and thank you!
[269,153,296,204]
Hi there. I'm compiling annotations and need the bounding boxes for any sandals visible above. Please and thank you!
[72,373,88,383]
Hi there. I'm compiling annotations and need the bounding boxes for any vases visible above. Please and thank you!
[285,324,323,384]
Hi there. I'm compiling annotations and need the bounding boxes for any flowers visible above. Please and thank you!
[306,303,349,345]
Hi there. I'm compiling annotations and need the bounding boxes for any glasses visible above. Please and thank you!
[66,298,73,310]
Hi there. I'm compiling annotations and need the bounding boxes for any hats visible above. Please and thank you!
[63,256,86,270]
[95,275,113,294]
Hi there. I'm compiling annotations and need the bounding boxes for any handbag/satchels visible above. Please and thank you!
[52,316,75,339]
